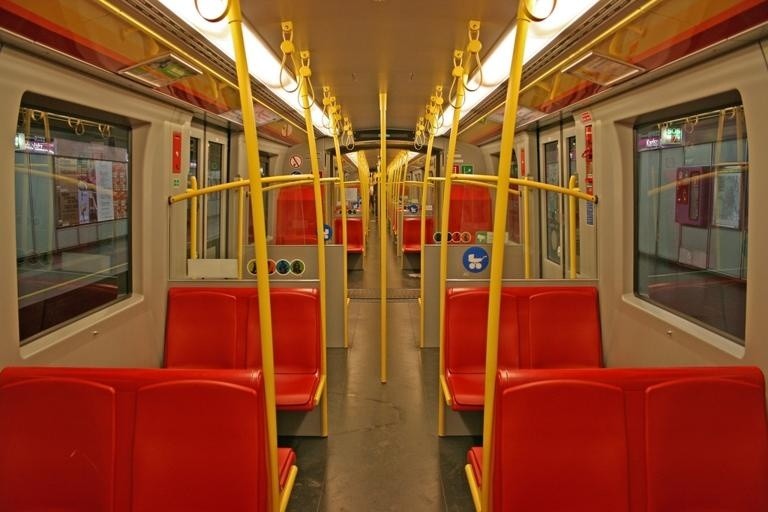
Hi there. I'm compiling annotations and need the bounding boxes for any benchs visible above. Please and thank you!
[333,217,363,254]
[402,218,433,254]
